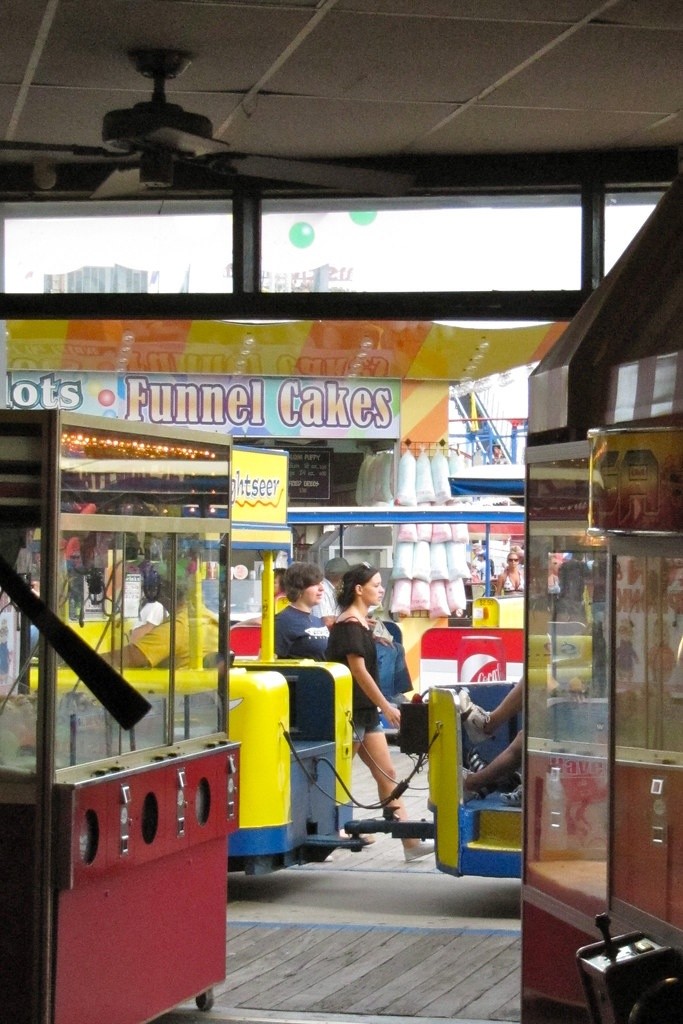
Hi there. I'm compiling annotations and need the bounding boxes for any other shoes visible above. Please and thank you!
[338,829,375,845]
[404,843,435,862]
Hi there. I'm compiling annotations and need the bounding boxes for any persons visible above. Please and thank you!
[488,446,502,464]
[274,562,331,659]
[496,552,524,596]
[31,574,40,646]
[313,557,414,705]
[100,556,218,670]
[510,547,584,592]
[326,563,435,861]
[558,562,589,623]
[469,543,486,580]
[459,672,591,802]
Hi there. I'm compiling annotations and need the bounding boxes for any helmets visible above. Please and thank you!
[146,550,193,596]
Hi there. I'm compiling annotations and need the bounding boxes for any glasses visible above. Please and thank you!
[362,561,372,570]
[508,559,519,562]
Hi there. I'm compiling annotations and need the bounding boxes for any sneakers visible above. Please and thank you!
[462,767,478,802]
[500,791,521,806]
[468,748,498,799]
[459,689,491,743]
